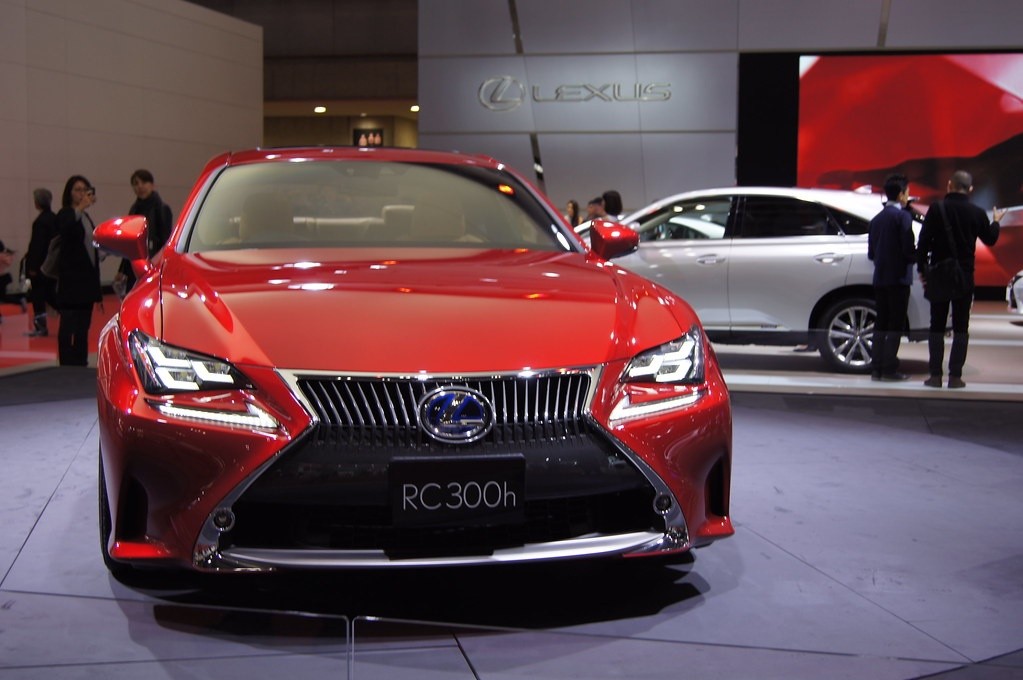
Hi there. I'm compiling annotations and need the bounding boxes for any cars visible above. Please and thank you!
[1006,269,1023,314]
[581,187,974,374]
[93,147,737,610]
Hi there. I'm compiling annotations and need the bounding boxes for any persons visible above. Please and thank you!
[583,189,623,225]
[112,169,172,304]
[868,175,917,380]
[917,171,1008,389]
[55,176,104,366]
[22,189,62,337]
[564,200,583,229]
[0,240,18,301]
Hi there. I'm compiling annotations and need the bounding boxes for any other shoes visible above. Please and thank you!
[882,370,912,380]
[925,374,942,388]
[871,370,883,380]
[24,329,49,336]
[949,377,966,387]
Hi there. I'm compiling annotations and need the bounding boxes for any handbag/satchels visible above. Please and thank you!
[40,235,62,278]
[924,259,965,303]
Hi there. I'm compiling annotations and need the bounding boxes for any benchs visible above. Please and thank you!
[206,206,416,242]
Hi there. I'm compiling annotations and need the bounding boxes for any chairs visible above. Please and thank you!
[220,194,296,245]
[397,196,481,245]
